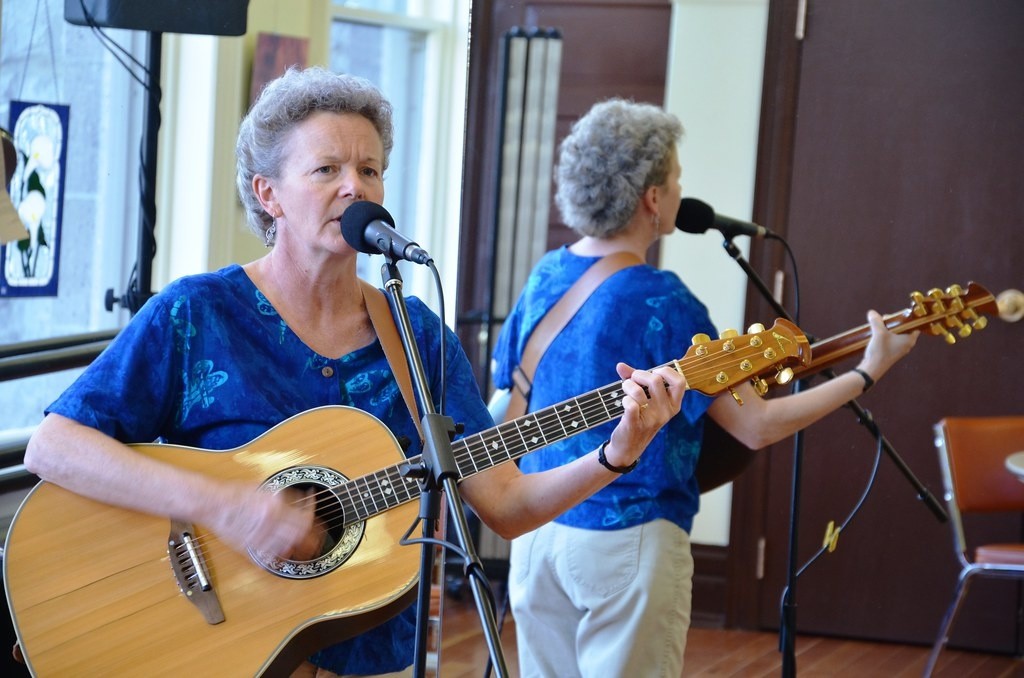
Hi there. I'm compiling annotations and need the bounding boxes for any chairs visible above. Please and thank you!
[922,419,1024,678]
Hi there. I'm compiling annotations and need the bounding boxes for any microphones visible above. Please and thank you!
[676,198,777,240]
[341,200,433,266]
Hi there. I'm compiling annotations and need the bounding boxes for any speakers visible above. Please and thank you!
[64,0,249,36]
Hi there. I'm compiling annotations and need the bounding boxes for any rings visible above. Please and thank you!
[641,403,648,409]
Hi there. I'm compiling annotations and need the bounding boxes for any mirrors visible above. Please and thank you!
[0,0,1024,678]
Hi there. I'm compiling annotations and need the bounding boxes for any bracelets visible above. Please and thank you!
[598,440,640,475]
[851,367,874,394]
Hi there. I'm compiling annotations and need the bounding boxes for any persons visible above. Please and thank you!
[490,101,920,678]
[24,64,688,678]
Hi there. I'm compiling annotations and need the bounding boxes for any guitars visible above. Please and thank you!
[3,318,814,678]
[694,276,1000,493]
